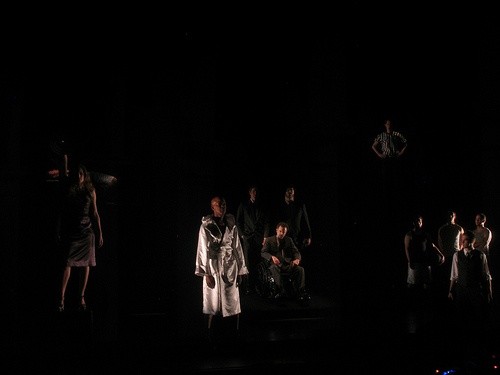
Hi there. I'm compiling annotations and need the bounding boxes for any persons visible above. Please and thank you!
[236,184,311,301]
[404,209,492,303]
[56,140,103,311]
[194,196,249,330]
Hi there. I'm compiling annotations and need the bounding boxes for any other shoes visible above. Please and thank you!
[79,296,88,310]
[58,299,65,312]
